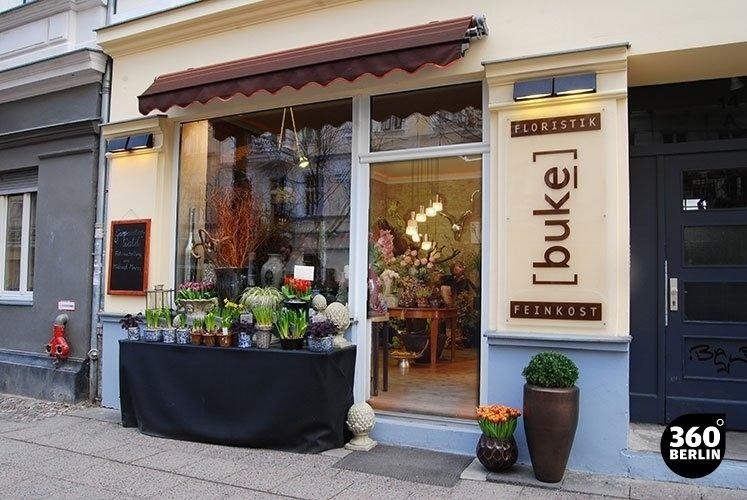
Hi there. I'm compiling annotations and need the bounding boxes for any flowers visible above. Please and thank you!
[474,403,521,439]
[282,277,312,300]
[174,281,218,305]
[369,230,463,314]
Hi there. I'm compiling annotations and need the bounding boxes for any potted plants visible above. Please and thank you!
[456,310,480,348]
[521,350,579,482]
[119,286,340,353]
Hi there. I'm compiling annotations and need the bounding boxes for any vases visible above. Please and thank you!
[475,435,518,471]
[261,254,288,289]
[215,267,248,317]
[400,334,448,366]
[184,207,196,282]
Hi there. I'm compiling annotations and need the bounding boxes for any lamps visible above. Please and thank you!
[290,107,310,169]
[126,134,153,149]
[553,73,597,96]
[405,157,443,251]
[106,136,128,152]
[512,78,554,101]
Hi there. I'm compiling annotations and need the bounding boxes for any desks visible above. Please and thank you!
[387,307,458,369]
[118,339,356,454]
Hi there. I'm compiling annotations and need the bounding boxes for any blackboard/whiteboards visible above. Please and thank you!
[107,219,151,296]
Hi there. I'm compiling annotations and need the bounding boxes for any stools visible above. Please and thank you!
[369,316,390,396]
